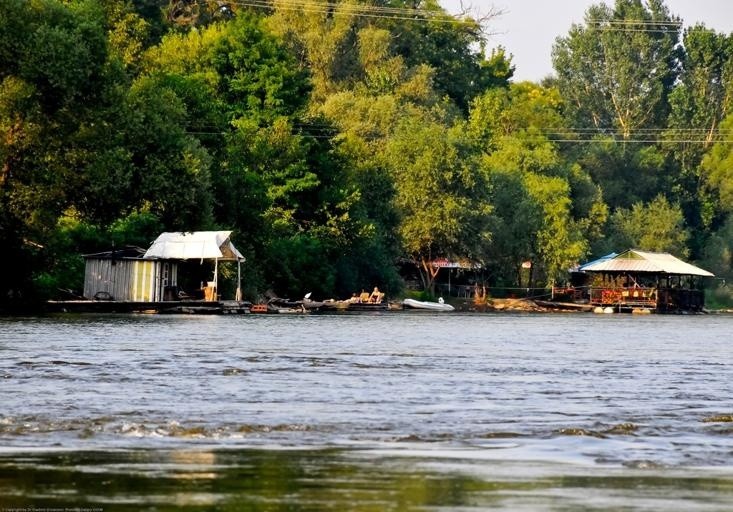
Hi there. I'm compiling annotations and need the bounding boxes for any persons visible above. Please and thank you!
[368,287,381,304]
[350,293,360,304]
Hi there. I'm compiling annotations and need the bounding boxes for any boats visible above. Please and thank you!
[400,296,456,312]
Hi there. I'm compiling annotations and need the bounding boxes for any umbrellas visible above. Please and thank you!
[430,251,481,296]
